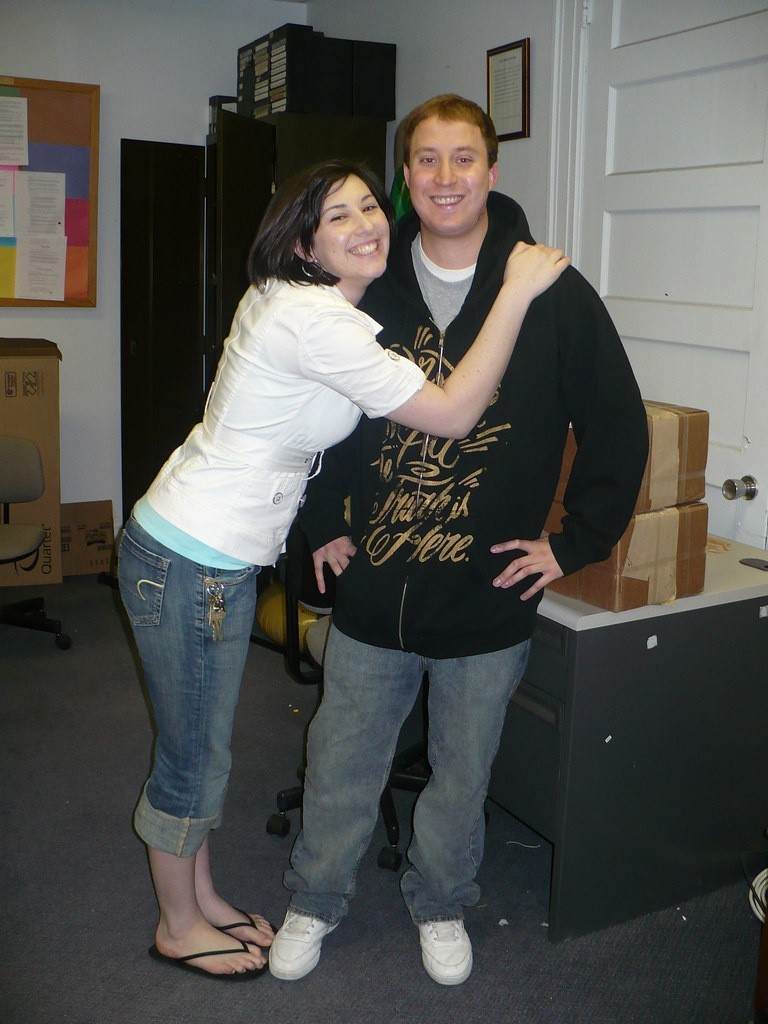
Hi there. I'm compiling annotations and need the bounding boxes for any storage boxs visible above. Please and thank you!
[236,23,396,123]
[543,398,708,614]
[0,338,62,585]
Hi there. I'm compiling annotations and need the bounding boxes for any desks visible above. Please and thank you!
[487,531,768,944]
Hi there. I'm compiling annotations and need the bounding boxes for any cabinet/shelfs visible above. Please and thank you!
[120,109,386,528]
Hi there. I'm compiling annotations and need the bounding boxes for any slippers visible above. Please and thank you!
[216,909,277,948]
[149,936,269,983]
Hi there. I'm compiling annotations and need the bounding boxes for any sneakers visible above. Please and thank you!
[269,905,341,981]
[421,920,473,985]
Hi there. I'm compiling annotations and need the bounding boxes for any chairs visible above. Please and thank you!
[0,434,72,650]
[267,512,430,871]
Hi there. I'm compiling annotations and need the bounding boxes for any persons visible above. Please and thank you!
[116,159,571,984]
[270,92,653,986]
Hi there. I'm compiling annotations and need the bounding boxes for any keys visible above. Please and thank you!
[207,594,228,642]
